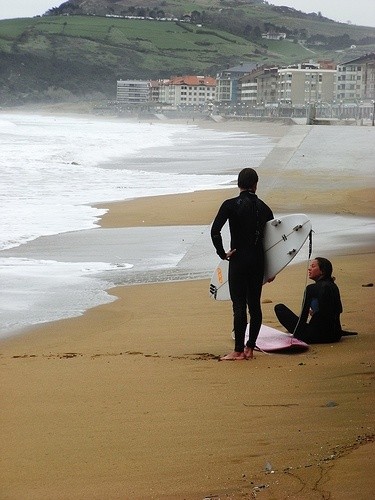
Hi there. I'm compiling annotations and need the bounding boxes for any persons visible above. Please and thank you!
[272,256,344,345]
[209,168,275,362]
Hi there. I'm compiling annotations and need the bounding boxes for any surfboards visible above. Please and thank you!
[209,214,313,300]
[231,323,310,353]
